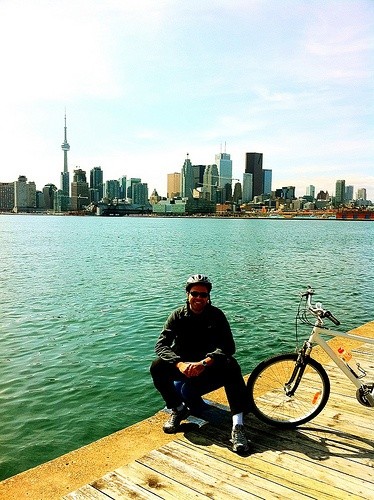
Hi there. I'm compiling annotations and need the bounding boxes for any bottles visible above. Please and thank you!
[338,348,364,377]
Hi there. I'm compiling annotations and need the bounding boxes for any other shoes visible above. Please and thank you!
[231,423,249,451]
[162,405,190,432]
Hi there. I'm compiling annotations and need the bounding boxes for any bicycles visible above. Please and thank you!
[245,285,374,428]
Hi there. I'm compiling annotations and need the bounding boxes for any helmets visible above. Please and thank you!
[186,273,212,291]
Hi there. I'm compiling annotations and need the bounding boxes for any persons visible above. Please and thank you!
[149,274,252,452]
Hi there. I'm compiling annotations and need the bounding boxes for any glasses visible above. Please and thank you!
[190,291,208,298]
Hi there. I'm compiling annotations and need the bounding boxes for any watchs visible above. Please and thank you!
[201,360,208,368]
